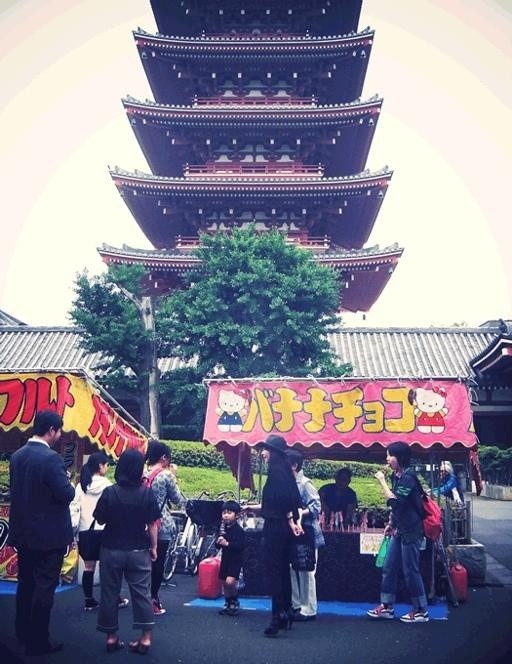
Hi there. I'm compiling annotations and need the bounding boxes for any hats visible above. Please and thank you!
[256,433,287,455]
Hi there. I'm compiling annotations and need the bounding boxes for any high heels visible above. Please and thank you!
[264,610,288,635]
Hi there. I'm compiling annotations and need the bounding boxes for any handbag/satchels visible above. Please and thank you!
[287,509,317,572]
[79,518,103,560]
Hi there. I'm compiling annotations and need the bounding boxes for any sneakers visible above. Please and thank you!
[365,603,395,620]
[293,609,317,621]
[400,609,430,623]
[128,639,150,654]
[285,607,296,632]
[117,596,130,607]
[84,600,101,611]
[24,639,66,655]
[220,603,241,616]
[152,600,167,615]
[106,638,124,651]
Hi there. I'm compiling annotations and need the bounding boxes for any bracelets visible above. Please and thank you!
[288,516,293,520]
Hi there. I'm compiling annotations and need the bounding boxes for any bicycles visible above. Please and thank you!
[162,487,258,582]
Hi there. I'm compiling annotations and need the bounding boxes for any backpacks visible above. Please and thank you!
[406,473,443,541]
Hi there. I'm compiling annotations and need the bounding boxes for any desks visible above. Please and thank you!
[237,527,437,605]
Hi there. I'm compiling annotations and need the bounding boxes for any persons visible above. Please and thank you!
[9,409,75,657]
[92,447,161,655]
[140,440,182,616]
[425,461,464,504]
[72,451,129,612]
[284,449,326,622]
[214,500,244,616]
[255,434,304,635]
[317,467,358,526]
[366,440,429,623]
